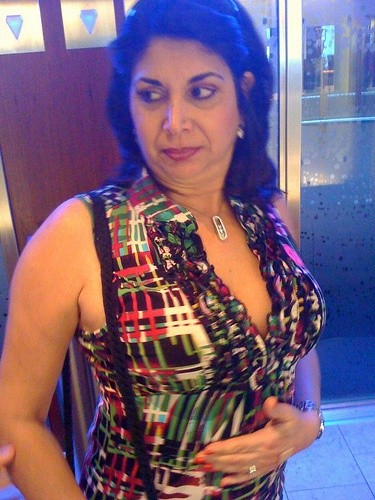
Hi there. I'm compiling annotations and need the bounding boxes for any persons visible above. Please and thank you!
[0,0,330,500]
[0,443,17,491]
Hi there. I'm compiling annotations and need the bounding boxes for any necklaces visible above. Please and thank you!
[163,194,229,241]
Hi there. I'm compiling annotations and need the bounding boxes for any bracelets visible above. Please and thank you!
[294,400,326,441]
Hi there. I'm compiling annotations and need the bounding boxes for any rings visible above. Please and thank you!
[249,464,256,474]
